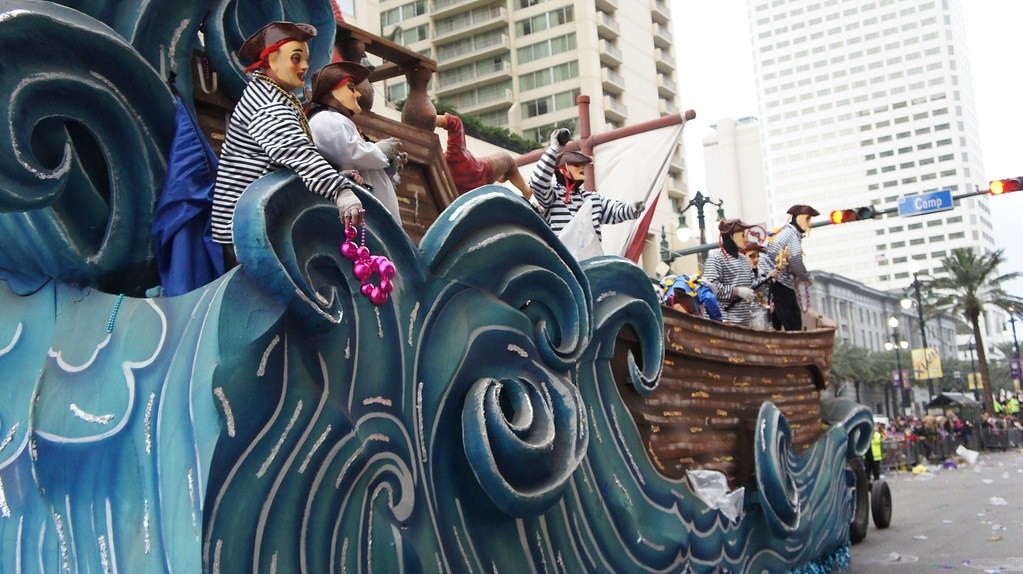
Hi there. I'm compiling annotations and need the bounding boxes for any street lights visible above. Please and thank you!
[1001,305,1023,393]
[899,274,934,405]
[963,343,980,401]
[677,192,725,269]
[884,315,910,416]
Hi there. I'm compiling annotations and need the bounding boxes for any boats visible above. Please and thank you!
[206,17,835,500]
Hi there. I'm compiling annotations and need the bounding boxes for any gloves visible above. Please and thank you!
[549,128,571,154]
[630,200,646,218]
[736,287,756,303]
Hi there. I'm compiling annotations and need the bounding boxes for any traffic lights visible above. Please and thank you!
[830,204,878,225]
[988,176,1022,195]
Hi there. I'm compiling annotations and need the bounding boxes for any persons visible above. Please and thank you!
[307,61,408,230]
[531,128,645,263]
[702,219,780,327]
[871,401,973,482]
[434,112,533,201]
[212,22,365,274]
[757,204,821,331]
[740,239,766,307]
[982,395,1023,453]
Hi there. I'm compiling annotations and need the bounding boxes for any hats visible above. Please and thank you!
[739,242,768,255]
[237,20,317,64]
[787,204,821,217]
[554,150,592,168]
[718,218,756,234]
[311,61,370,102]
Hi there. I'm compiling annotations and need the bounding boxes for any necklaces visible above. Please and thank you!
[795,279,810,313]
